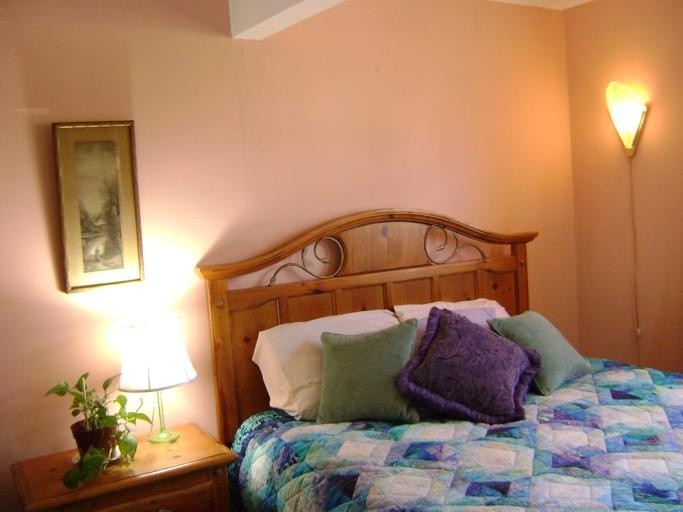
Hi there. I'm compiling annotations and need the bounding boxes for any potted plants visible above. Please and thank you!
[46,371,154,488]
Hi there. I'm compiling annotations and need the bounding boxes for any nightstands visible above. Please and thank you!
[9,423,237,510]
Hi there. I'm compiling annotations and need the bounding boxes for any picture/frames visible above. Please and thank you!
[51,119,144,295]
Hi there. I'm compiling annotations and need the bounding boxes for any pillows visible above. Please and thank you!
[409,308,496,362]
[484,310,595,394]
[397,305,541,423]
[252,308,400,418]
[315,316,421,422]
[392,297,511,320]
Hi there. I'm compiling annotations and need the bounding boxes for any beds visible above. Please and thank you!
[197,210,681,509]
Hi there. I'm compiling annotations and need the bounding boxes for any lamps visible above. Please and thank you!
[117,313,197,442]
[603,82,648,159]
